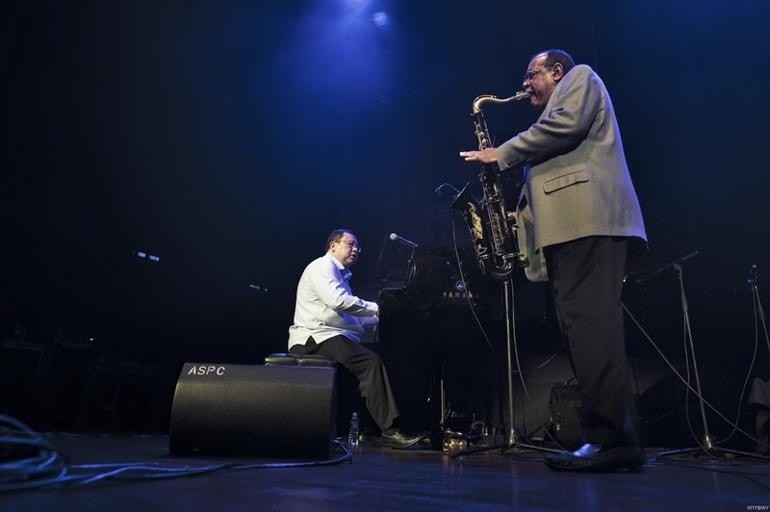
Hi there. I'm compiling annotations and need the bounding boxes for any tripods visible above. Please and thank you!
[659,274,770,461]
[448,282,573,459]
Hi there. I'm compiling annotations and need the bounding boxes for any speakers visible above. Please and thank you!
[168,362,337,463]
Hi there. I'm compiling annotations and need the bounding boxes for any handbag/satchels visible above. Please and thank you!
[546,376,640,449]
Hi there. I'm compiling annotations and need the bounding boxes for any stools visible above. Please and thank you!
[264,349,339,371]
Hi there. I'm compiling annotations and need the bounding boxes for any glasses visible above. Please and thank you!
[524,63,553,82]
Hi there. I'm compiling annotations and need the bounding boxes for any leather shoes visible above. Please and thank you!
[382,433,427,450]
[544,444,645,474]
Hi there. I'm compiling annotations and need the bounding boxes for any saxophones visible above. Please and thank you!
[448,92,534,281]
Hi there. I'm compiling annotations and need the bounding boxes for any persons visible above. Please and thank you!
[459,48,651,473]
[288,229,425,449]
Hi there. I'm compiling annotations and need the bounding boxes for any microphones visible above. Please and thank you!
[384,230,418,249]
